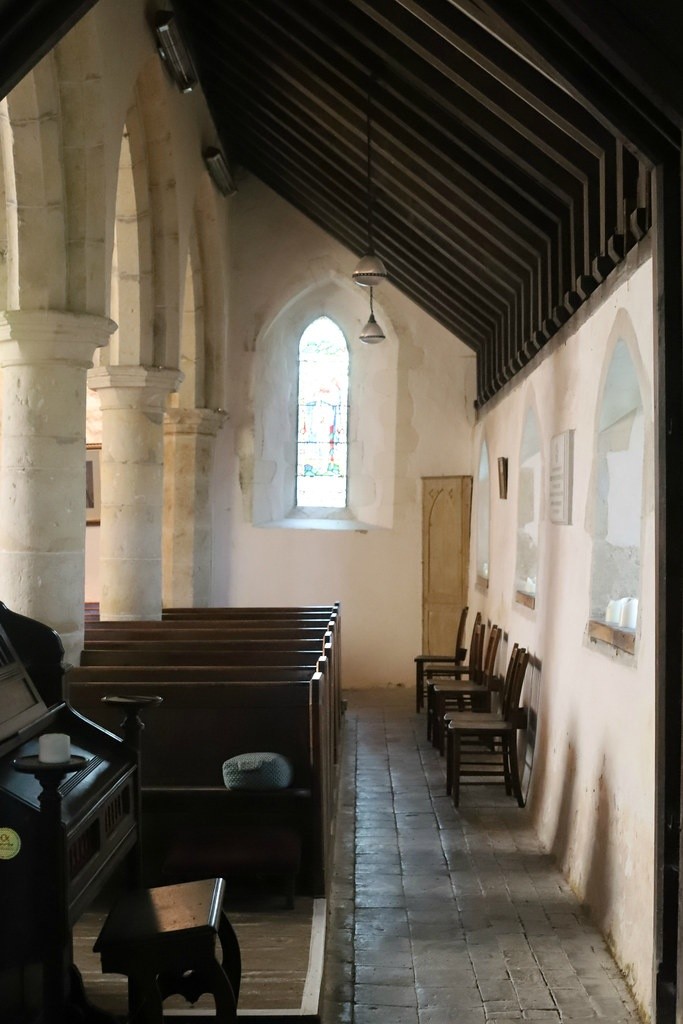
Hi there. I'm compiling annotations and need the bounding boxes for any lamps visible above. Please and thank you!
[354,81,387,344]
[155,9,199,94]
[204,145,238,198]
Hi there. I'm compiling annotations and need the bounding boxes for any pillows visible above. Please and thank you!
[221,753,294,791]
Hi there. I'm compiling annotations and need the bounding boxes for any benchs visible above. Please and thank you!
[68,601,341,911]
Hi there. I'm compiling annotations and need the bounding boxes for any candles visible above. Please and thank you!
[39,733,70,763]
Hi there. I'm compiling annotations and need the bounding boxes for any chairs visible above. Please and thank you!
[413,605,529,811]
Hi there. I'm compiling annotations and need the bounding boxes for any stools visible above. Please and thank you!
[92,878,242,1024]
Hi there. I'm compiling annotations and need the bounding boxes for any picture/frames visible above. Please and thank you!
[86,443,102,527]
